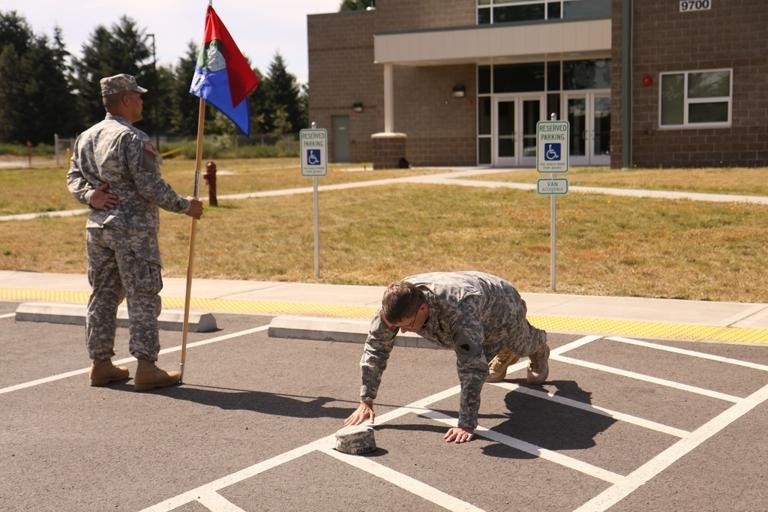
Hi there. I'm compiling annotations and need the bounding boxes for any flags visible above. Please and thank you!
[190,5,259,141]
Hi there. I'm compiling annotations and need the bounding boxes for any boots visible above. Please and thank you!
[526,344,550,385]
[134,358,181,391]
[90,358,130,387]
[484,347,520,383]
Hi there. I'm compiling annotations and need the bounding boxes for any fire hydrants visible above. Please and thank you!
[203,161,219,206]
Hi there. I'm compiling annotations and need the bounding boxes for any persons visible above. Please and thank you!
[66,74,202,389]
[342,269,551,444]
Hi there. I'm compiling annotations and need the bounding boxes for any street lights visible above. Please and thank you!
[144,31,162,154]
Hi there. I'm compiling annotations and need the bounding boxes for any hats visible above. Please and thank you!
[99,73,148,96]
[334,423,378,455]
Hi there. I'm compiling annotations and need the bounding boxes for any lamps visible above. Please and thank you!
[351,101,364,113]
[451,85,466,99]
